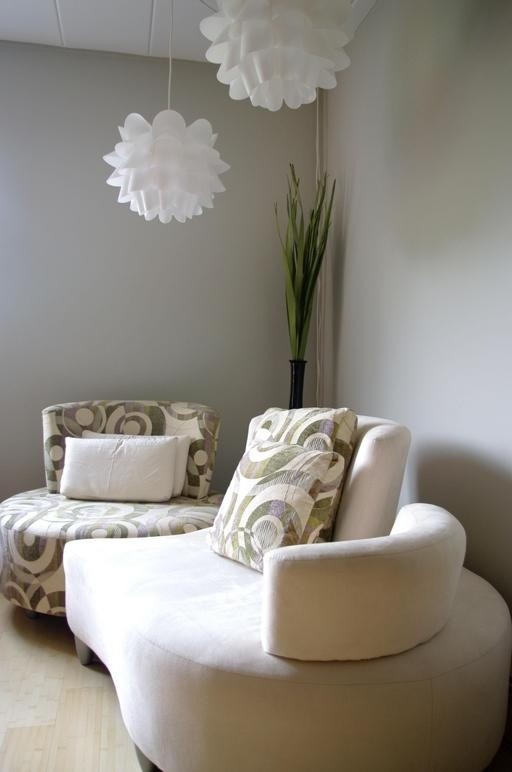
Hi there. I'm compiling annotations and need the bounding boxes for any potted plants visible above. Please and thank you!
[265,158,336,412]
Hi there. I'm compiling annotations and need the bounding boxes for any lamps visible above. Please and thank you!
[198,1,357,111]
[105,2,230,223]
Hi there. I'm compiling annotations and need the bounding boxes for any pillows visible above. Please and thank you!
[60,436,178,503]
[245,408,357,543]
[209,428,344,573]
[81,430,191,497]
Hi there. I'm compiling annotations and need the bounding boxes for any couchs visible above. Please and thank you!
[1,396,224,618]
[64,407,511,772]
